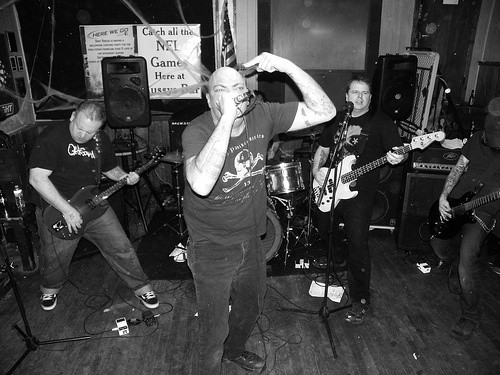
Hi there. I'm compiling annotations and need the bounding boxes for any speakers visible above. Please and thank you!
[70,181,131,261]
[368,163,406,235]
[101,55,152,129]
[371,53,418,121]
[397,172,449,252]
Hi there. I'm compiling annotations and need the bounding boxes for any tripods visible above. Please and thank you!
[275,119,353,359]
[0,236,92,375]
[127,128,164,235]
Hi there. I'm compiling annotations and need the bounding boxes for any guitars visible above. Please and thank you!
[42,150,162,240]
[427,191,499,240]
[312,130,446,212]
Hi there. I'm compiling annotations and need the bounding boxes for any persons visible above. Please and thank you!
[180,51,337,375]
[434,97,500,340]
[28,101,159,310]
[312,80,407,324]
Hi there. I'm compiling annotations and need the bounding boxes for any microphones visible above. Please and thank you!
[234,90,253,106]
[343,101,354,125]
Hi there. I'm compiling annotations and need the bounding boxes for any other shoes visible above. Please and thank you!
[229,351,266,371]
[455,319,476,337]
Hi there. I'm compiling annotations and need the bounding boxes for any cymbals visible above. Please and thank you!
[144,151,183,164]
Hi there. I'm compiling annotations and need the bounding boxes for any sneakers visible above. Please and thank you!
[344,297,370,325]
[313,258,346,267]
[135,290,159,309]
[41,294,58,310]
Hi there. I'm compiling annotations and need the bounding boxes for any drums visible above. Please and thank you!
[265,161,305,196]
[260,206,285,263]
